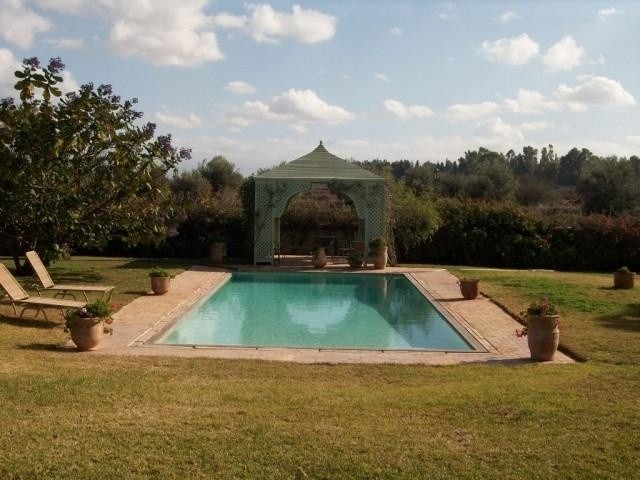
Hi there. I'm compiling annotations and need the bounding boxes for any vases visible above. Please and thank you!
[457,279,482,299]
[527,315,561,361]
[70,317,101,350]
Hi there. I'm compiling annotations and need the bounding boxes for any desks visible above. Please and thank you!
[352,240,365,256]
[319,235,344,257]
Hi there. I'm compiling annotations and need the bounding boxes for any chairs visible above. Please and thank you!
[337,239,355,262]
[0,262,88,326]
[25,251,115,305]
[322,239,335,264]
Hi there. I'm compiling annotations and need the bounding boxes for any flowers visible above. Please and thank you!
[526,297,561,314]
[60,300,113,335]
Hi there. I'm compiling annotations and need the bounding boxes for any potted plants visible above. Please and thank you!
[614,266,636,289]
[207,236,227,265]
[368,236,389,269]
[149,268,171,294]
[312,243,327,267]
